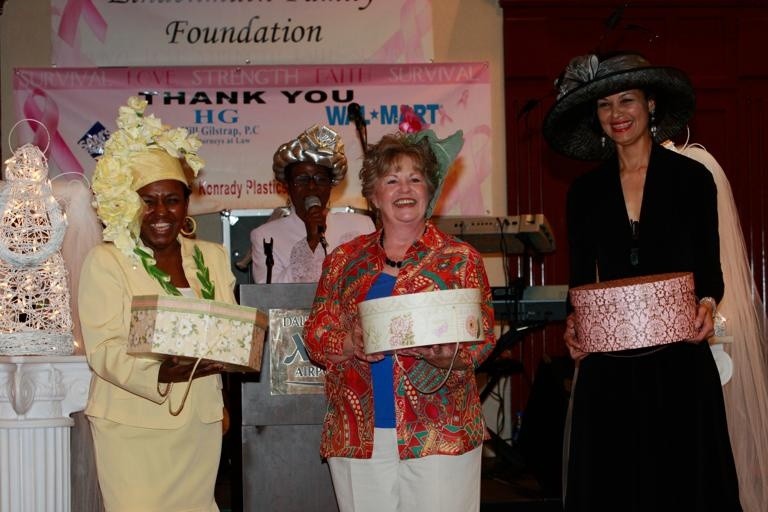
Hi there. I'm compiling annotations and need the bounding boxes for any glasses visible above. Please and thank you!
[287,173,334,188]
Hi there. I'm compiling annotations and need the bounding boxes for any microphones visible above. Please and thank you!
[347,102,360,122]
[304,194,327,249]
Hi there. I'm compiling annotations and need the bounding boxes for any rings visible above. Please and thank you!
[415,355,424,360]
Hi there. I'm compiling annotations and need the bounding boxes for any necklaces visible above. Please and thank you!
[380,227,427,267]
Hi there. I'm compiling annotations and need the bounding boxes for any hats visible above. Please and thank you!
[540,52,698,163]
[129,146,189,192]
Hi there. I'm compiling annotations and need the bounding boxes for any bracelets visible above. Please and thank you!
[699,297,721,319]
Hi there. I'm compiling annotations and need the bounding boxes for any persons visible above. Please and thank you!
[550,53,743,511]
[249,125,375,283]
[72,94,242,512]
[302,133,501,512]
